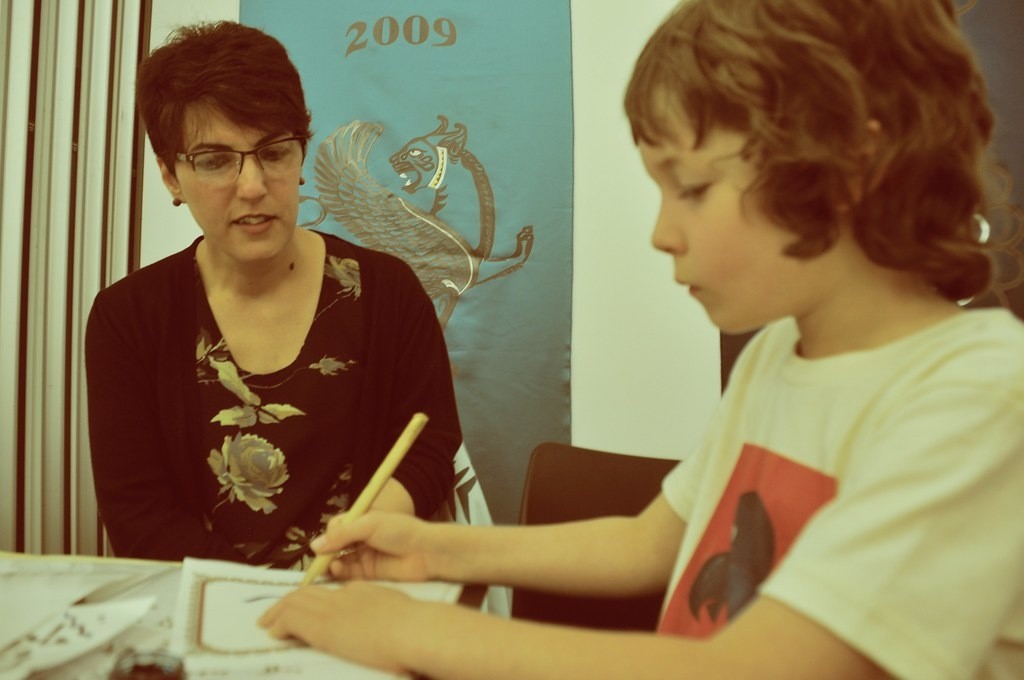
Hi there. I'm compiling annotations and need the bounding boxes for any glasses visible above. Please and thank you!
[172,136,307,186]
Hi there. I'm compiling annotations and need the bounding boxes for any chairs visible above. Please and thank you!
[511,441,682,634]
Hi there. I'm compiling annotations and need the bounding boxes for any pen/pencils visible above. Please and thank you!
[300,411,429,586]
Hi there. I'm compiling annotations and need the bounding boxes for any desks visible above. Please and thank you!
[0,552,488,680]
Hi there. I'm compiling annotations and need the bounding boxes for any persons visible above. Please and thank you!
[84,22,464,574]
[256,0,1024,680]
